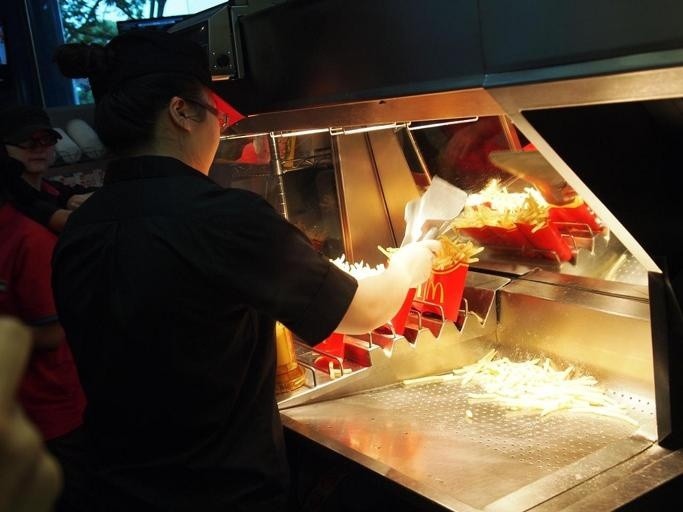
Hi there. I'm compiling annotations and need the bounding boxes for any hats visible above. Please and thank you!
[4,108,62,139]
[89,30,248,130]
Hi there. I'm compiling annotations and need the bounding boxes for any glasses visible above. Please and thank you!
[183,97,228,129]
[5,135,57,148]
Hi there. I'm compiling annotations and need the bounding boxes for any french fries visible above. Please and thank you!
[453,188,584,234]
[329,229,484,286]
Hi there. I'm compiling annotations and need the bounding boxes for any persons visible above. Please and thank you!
[0,108,93,512]
[52,26,441,512]
